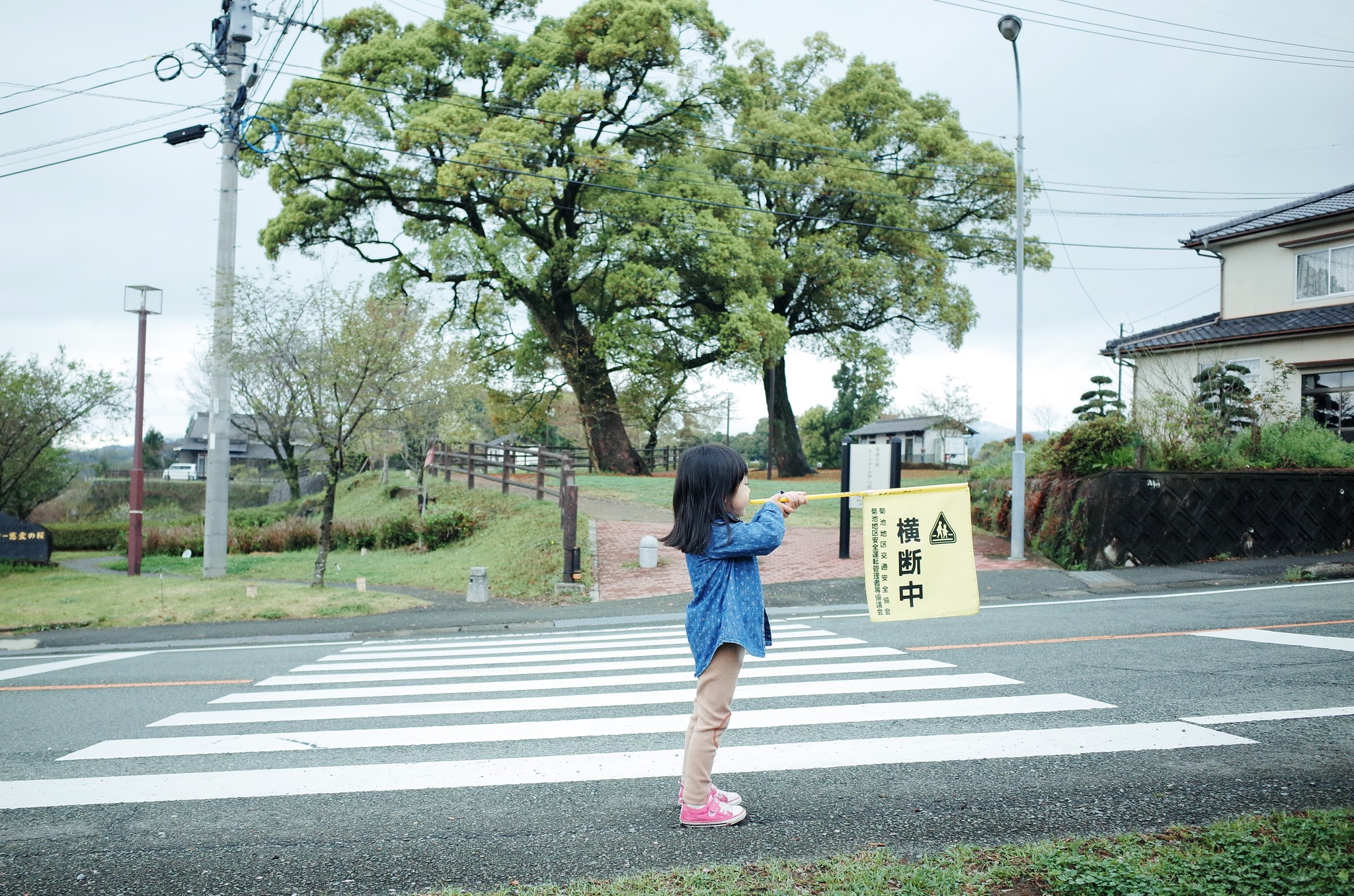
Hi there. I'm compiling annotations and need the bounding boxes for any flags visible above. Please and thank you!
[863,487,980,621]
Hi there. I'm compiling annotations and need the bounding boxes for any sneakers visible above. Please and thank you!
[679,793,746,828]
[678,783,742,806]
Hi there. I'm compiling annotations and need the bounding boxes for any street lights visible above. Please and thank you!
[997,13,1027,567]
[123,284,162,577]
[546,408,555,467]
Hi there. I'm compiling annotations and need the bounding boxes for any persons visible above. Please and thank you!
[656,445,807,827]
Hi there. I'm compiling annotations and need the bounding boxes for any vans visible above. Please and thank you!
[162,462,198,481]
